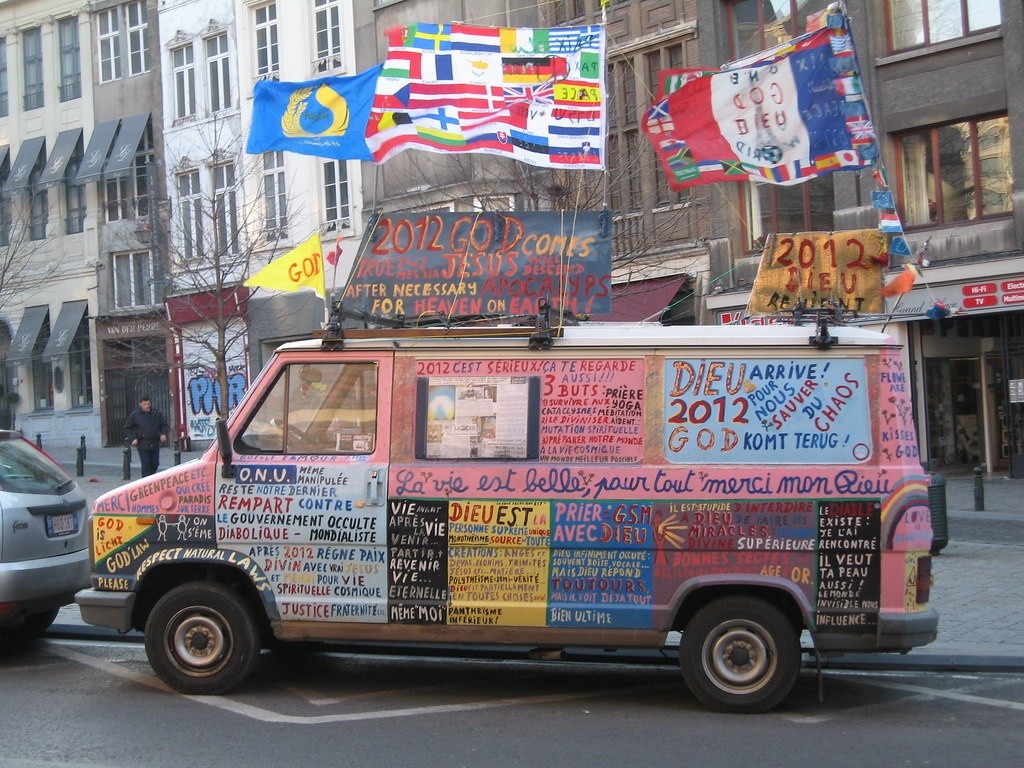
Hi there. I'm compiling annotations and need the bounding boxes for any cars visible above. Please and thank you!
[0,428,93,653]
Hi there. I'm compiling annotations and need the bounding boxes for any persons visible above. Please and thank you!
[124,396,167,478]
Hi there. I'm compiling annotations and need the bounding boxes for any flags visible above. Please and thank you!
[246,64,384,160]
[364,20,608,174]
[642,11,918,296]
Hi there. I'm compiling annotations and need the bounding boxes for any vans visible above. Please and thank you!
[75,297,937,714]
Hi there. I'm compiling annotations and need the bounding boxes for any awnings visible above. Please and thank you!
[102,112,152,181]
[38,128,83,189]
[5,304,48,363]
[74,119,122,187]
[0,136,45,191]
[42,300,89,363]
